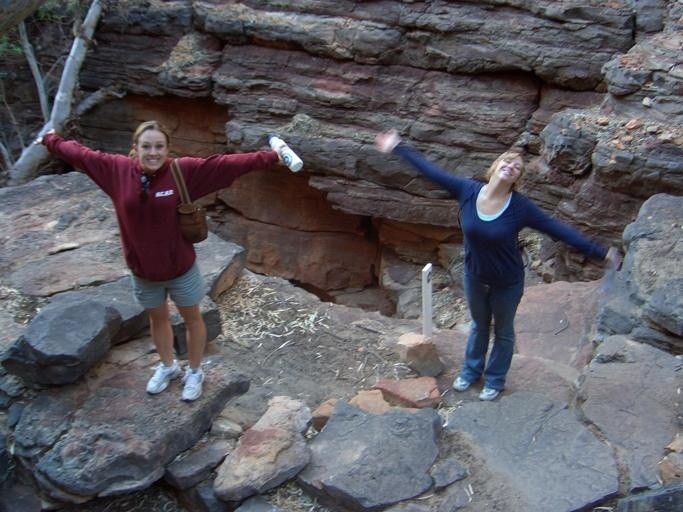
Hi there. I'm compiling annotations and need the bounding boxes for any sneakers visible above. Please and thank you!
[479,386,499,400]
[453,377,470,392]
[146,359,205,401]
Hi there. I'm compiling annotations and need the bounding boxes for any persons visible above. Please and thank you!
[374,128,618,401]
[33,120,285,401]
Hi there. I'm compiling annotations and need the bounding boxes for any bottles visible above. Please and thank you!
[265,133,304,173]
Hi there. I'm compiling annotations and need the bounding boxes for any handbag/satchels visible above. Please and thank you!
[170,158,208,243]
